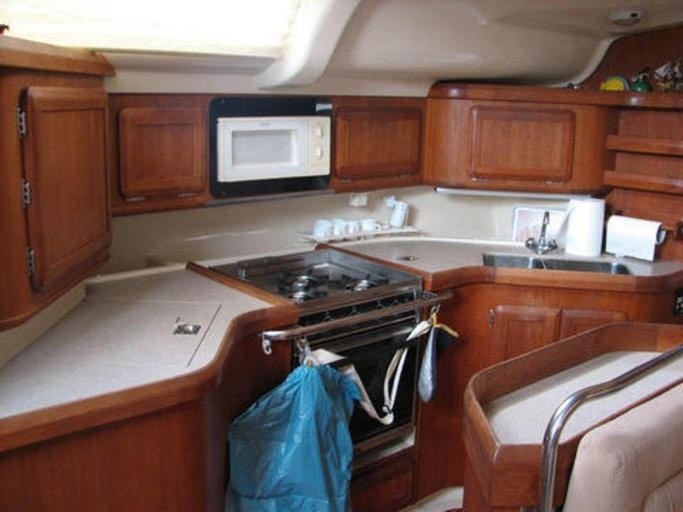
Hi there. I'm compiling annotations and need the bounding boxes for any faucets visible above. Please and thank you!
[525,210,557,255]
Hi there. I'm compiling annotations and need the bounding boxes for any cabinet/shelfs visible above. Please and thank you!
[464,320,683,512]
[0,39,116,328]
[320,238,683,505]
[422,40,683,260]
[113,90,208,215]
[333,92,425,192]
[1,262,301,510]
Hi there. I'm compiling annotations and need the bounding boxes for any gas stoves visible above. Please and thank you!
[210,249,420,303]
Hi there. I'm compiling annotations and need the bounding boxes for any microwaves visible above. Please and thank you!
[209,97,332,200]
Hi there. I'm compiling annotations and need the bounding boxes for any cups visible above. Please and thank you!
[312,218,382,240]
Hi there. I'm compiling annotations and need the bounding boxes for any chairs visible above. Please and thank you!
[541,341,683,512]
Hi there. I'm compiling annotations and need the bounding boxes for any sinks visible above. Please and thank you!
[541,257,634,276]
[481,251,546,270]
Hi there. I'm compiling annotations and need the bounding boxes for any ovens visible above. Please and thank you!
[289,289,422,473]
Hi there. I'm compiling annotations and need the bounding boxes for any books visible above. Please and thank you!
[512,207,566,243]
